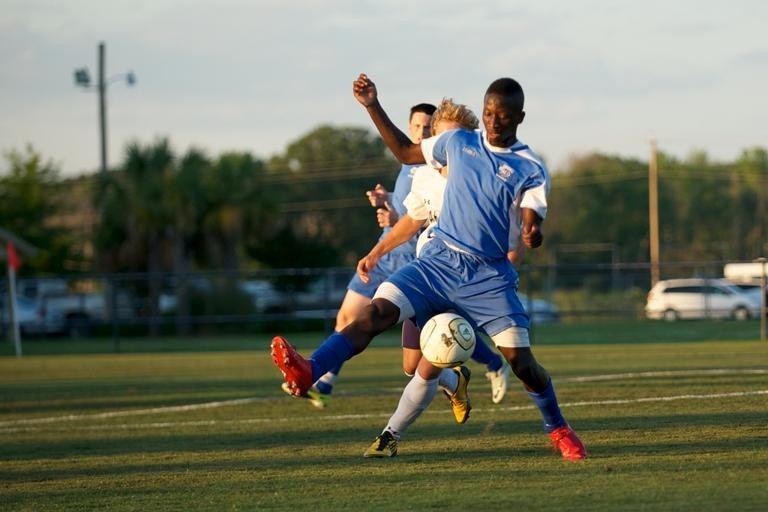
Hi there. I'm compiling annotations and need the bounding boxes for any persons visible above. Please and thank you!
[354,94,481,458]
[269,72,587,460]
[280,101,512,410]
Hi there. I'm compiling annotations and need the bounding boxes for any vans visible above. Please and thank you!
[643,276,760,322]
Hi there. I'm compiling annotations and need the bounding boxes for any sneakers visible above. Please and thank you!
[549,424,586,461]
[486,355,511,404]
[271,336,331,409]
[363,431,400,459]
[443,366,471,423]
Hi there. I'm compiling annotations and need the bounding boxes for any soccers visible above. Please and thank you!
[419,312,477,368]
